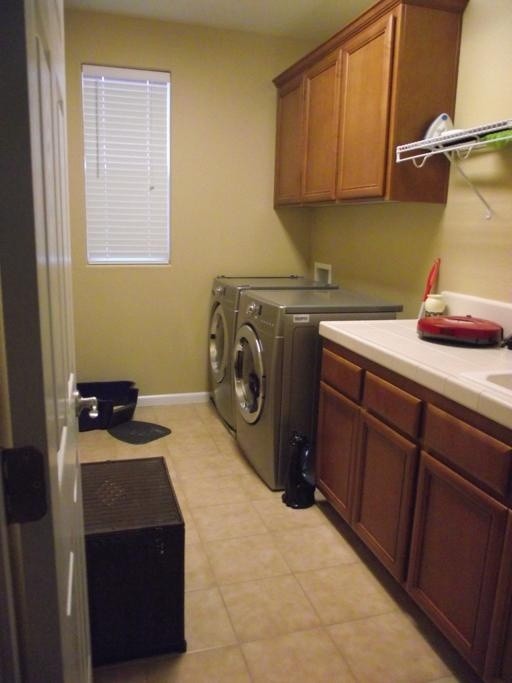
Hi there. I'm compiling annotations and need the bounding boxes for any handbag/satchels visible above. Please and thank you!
[78,379,138,431]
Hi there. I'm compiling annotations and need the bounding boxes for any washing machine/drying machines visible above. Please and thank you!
[236,285,404,490]
[206,276,337,433]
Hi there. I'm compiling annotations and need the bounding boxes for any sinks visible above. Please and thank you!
[486,371,512,392]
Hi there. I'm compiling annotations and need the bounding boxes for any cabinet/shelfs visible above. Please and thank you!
[302,49,340,206]
[339,5,468,205]
[272,54,302,207]
[407,392,510,683]
[318,344,424,585]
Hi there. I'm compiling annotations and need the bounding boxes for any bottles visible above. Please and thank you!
[426,293,445,315]
[284,432,317,508]
